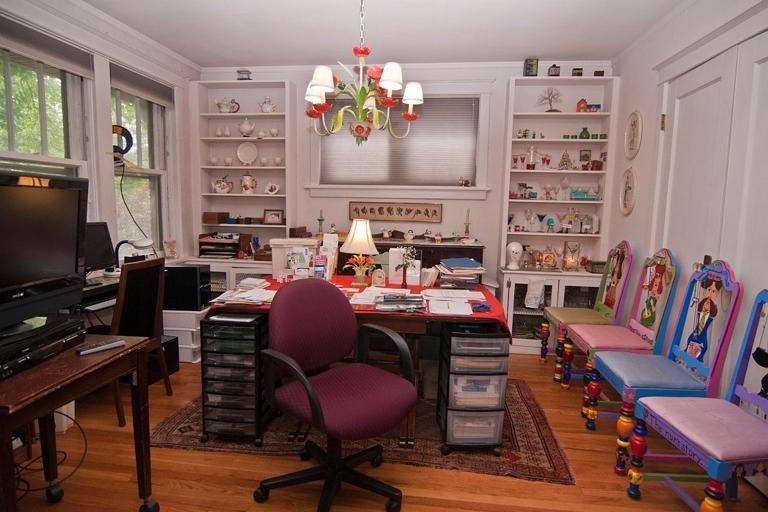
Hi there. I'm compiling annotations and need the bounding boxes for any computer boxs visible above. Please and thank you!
[162,265,211,310]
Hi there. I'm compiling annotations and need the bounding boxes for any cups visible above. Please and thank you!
[555,211,567,232]
[543,182,551,199]
[257,128,266,138]
[567,207,574,222]
[551,183,560,200]
[224,157,233,166]
[314,264,324,280]
[575,214,588,234]
[536,211,547,232]
[215,127,221,137]
[591,183,601,200]
[286,269,294,283]
[529,191,538,200]
[571,135,577,139]
[211,155,219,165]
[592,134,598,138]
[260,157,267,167]
[270,128,279,137]
[568,185,579,201]
[580,185,591,200]
[523,208,532,232]
[600,133,606,139]
[559,176,570,200]
[563,135,569,139]
[273,157,281,166]
[224,127,231,136]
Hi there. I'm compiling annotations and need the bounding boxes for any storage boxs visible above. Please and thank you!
[162,305,212,363]
[35,399,76,434]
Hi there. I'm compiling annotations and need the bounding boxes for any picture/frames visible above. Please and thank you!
[620,167,636,215]
[264,209,283,224]
[349,201,442,223]
[624,111,642,160]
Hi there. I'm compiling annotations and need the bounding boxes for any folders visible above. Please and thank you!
[441,257,483,269]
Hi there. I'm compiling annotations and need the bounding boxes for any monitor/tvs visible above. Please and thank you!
[0,165,90,337]
[83,221,118,285]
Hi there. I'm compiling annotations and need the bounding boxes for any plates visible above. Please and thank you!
[237,142,258,162]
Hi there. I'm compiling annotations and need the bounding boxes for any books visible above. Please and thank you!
[421,257,489,288]
[235,277,271,291]
[210,289,278,306]
[375,292,428,312]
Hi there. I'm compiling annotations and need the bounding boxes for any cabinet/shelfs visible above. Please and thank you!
[187,80,298,298]
[496,77,621,337]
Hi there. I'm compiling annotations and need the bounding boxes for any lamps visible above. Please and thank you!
[304,0,425,146]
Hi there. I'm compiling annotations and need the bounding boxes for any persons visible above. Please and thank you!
[640,263,667,327]
[603,252,625,307]
[686,276,724,363]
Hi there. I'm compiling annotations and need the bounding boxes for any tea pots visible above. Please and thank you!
[215,97,240,113]
[237,116,256,137]
[257,95,277,113]
[240,170,257,195]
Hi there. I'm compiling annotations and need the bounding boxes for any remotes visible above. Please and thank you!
[74,338,127,357]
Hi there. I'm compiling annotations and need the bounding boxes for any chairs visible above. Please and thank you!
[586,260,745,476]
[85,257,172,427]
[252,278,417,512]
[627,288,768,512]
[541,240,634,384]
[562,248,680,418]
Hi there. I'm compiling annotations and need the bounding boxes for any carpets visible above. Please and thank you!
[149,360,575,485]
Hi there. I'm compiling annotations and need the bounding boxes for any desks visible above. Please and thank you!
[200,275,511,456]
[82,264,169,313]
[308,236,487,284]
[0,336,159,512]
